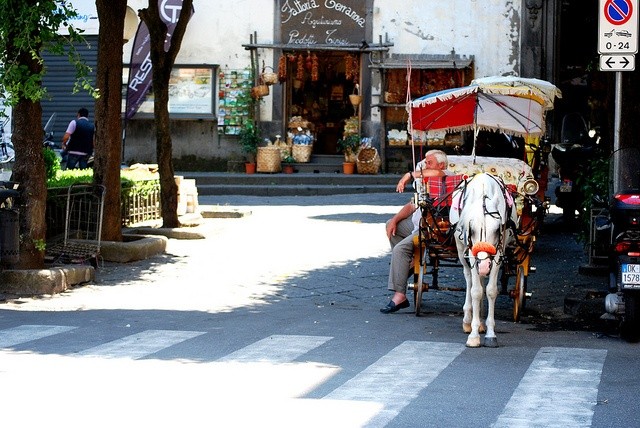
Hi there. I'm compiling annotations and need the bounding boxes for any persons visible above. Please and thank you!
[380,149,448,317]
[62,107,95,169]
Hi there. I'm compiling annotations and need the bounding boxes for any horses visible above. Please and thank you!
[450,172,517,350]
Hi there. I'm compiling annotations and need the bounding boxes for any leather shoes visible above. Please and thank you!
[380,299,410,313]
[408,266,415,278]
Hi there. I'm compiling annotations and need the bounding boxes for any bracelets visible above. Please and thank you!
[409,170,414,185]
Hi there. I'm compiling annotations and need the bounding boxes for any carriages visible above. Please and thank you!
[406,74,562,348]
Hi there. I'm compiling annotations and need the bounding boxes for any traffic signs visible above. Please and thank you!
[597,0,639,54]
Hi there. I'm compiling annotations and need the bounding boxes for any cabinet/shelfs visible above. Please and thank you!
[380,69,477,176]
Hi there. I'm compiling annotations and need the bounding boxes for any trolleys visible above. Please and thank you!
[45,182,107,270]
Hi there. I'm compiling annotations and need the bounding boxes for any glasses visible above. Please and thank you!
[424,160,435,165]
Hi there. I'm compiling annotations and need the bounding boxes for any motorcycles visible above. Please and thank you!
[42,112,95,169]
[552,127,602,225]
[596,147,640,343]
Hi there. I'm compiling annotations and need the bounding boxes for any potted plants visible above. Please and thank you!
[338,135,362,174]
[238,118,263,174]
[283,156,295,173]
